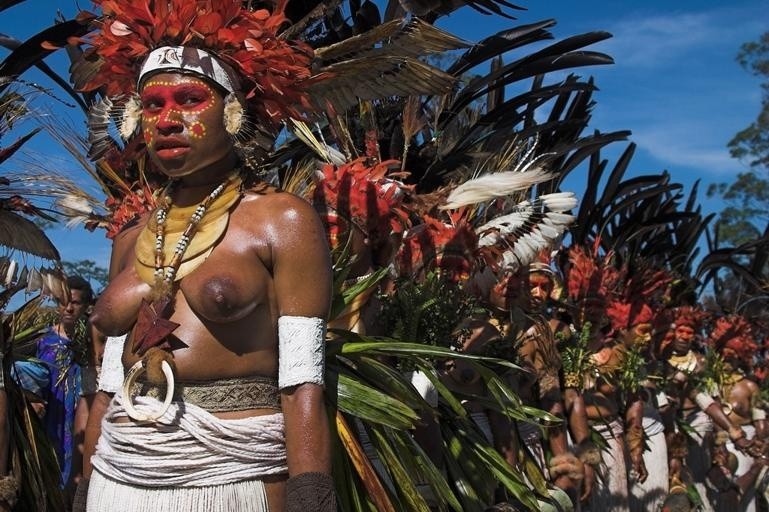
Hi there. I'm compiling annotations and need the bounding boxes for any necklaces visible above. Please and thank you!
[120,175,243,423]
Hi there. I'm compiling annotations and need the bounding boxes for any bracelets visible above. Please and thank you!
[71,476,91,511]
[285,472,337,511]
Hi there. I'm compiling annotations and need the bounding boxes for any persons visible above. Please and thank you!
[2,242,768,512]
[76,43,335,511]
